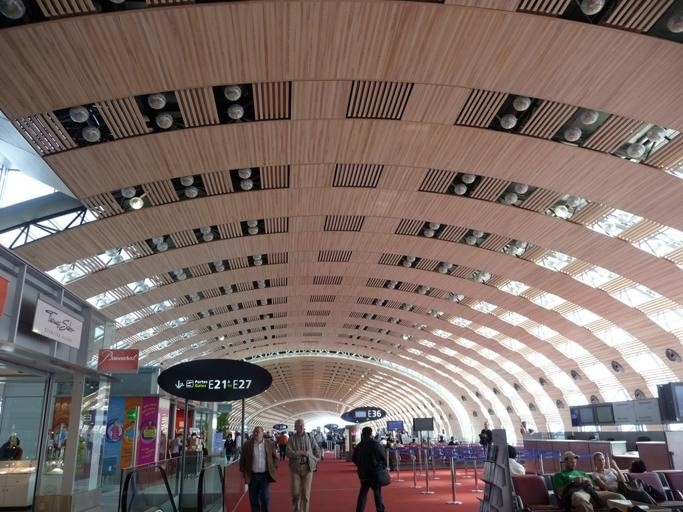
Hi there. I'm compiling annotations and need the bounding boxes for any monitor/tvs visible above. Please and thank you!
[387,421,404,431]
[413,418,433,431]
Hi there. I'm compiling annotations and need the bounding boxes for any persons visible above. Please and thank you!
[479,422,493,459]
[552,450,627,512]
[285,419,322,512]
[351,427,388,512]
[239,426,280,512]
[520,421,529,435]
[171,431,345,465]
[124,420,138,439]
[374,427,458,450]
[106,418,122,440]
[0,436,17,461]
[591,451,657,504]
[508,445,526,477]
[145,420,155,430]
[16,439,22,460]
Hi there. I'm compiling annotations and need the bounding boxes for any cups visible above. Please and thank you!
[576,477,583,484]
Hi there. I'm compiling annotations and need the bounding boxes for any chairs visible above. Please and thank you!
[389,444,484,469]
[512,467,682,511]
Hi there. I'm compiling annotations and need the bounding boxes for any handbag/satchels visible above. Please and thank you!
[360,466,391,487]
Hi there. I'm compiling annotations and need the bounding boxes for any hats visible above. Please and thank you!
[563,450,580,460]
